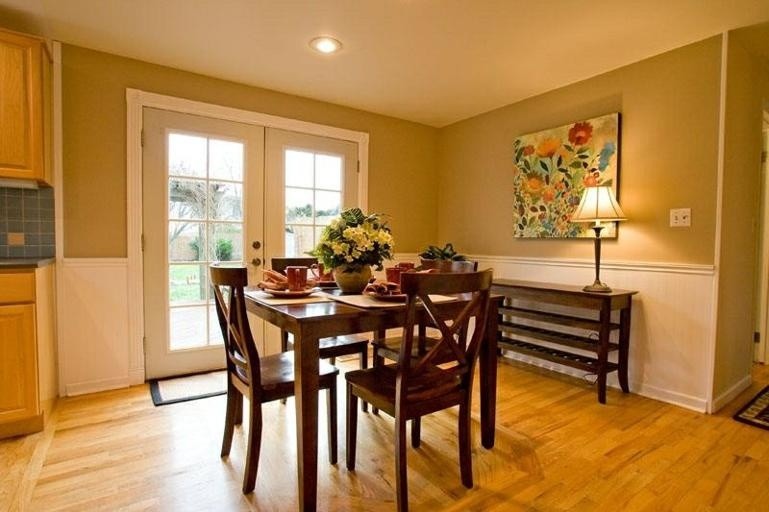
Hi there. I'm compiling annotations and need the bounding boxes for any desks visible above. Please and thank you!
[486,276,639,409]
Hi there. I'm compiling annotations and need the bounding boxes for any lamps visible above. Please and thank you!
[568,184,630,293]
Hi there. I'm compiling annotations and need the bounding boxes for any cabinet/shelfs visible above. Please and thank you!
[1,264,56,439]
[0,23,56,193]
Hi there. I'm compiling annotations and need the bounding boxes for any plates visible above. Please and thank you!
[264,288,316,298]
[371,292,408,303]
[315,281,339,290]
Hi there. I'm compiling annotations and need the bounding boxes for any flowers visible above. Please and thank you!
[302,207,395,275]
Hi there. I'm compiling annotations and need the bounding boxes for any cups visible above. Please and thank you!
[310,262,333,281]
[385,264,407,282]
[395,262,415,269]
[285,264,309,292]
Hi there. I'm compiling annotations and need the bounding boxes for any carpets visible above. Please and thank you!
[148,364,230,407]
[732,384,768,431]
